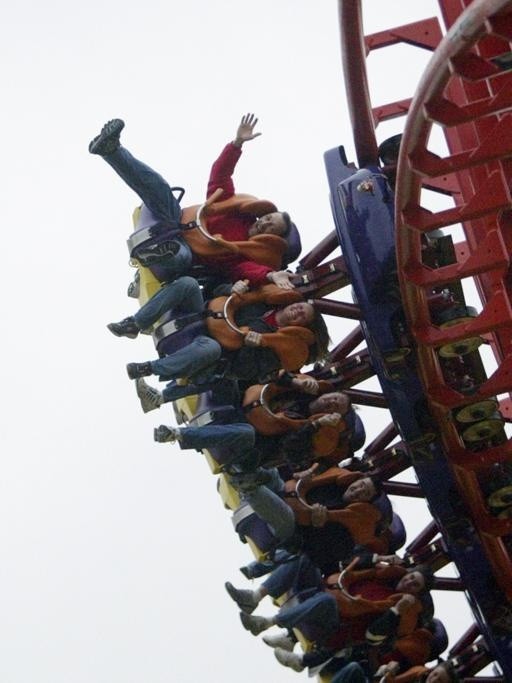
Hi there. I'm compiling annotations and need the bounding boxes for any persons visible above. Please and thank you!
[91,112,512,682]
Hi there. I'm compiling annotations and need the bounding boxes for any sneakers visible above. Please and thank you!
[134,240,180,263]
[89,118,125,157]
[135,377,162,413]
[273,646,305,672]
[230,470,272,493]
[240,565,254,579]
[262,633,294,653]
[240,612,269,637]
[153,424,176,443]
[106,315,140,339]
[225,581,259,614]
[126,360,152,380]
